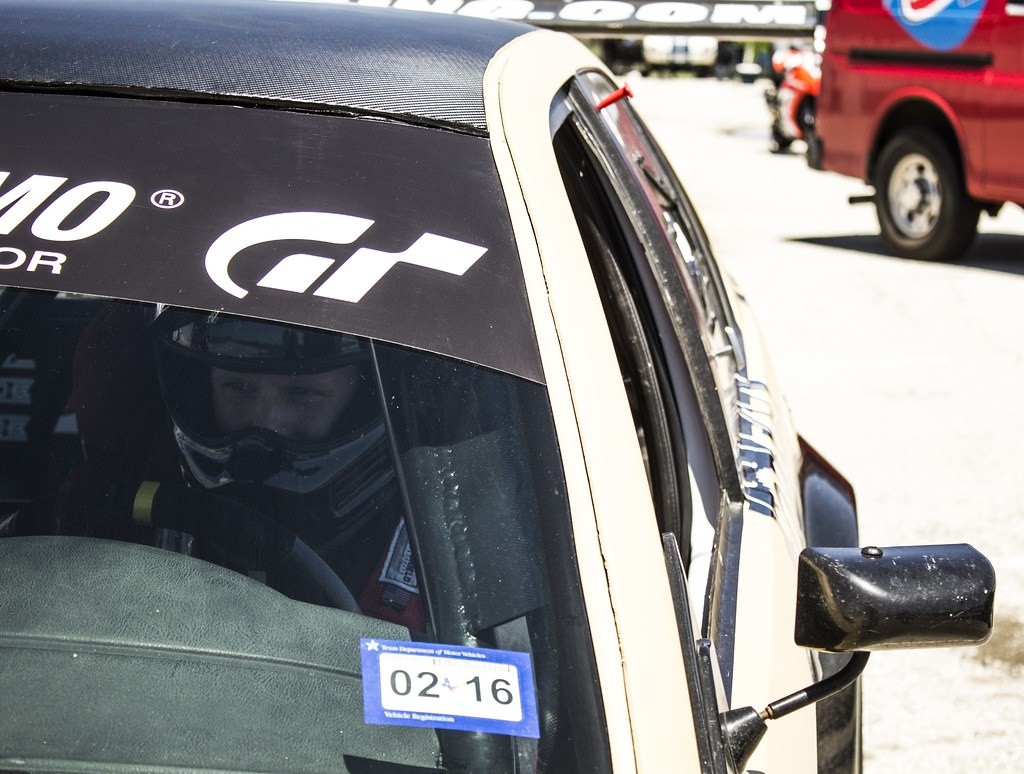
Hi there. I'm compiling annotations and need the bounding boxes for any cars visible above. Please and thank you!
[0,0,997,773]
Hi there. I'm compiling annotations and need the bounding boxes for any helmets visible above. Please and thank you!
[149,303,397,547]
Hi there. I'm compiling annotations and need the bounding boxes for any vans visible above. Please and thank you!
[764,0,1024,267]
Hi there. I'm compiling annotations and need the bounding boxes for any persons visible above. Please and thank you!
[99,312,402,618]
